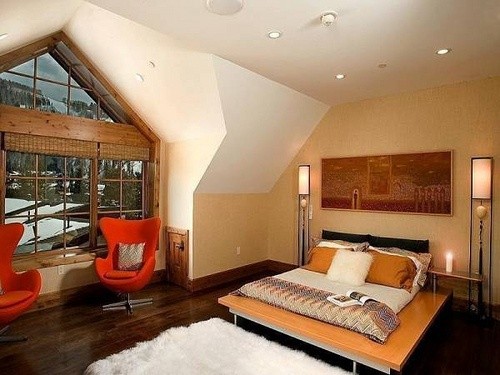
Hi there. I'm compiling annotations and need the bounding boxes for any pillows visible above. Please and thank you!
[365,250,417,293]
[118,242,145,270]
[328,249,372,286]
[303,248,354,273]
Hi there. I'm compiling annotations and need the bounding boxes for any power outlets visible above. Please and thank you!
[58,265,65,276]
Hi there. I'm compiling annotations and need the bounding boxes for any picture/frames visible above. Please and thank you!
[319,149,454,216]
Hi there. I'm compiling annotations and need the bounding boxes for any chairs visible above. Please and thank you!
[0,223,41,342]
[95,218,161,312]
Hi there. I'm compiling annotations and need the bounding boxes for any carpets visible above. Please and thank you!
[85,317,354,375]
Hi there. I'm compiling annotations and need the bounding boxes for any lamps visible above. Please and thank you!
[469,157,494,306]
[297,165,310,267]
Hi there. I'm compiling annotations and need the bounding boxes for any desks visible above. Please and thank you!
[427,266,483,293]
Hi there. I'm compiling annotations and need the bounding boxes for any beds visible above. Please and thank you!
[219,231,454,375]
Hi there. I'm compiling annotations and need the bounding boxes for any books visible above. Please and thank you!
[328,291,379,307]
